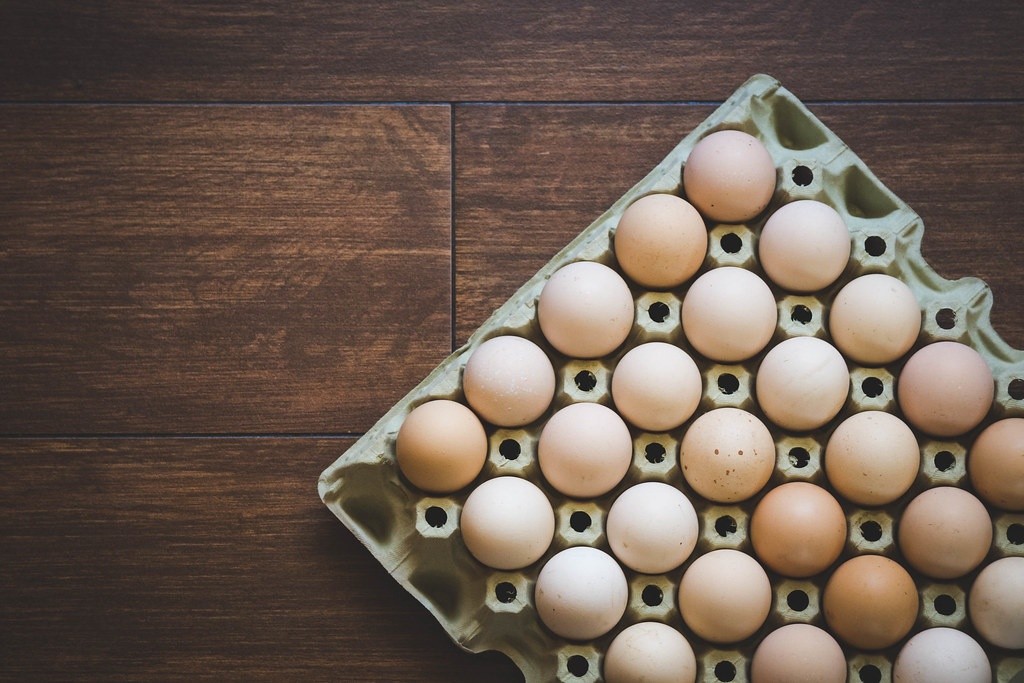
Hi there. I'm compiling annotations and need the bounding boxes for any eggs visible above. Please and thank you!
[396,129,1024,683]
